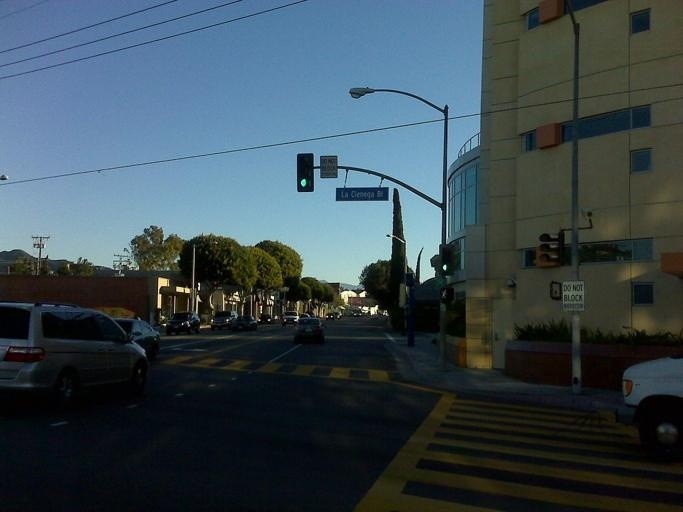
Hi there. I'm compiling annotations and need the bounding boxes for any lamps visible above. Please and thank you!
[505,274,517,286]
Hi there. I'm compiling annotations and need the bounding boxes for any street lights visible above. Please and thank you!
[383,232,406,299]
[191,240,219,310]
[346,84,454,369]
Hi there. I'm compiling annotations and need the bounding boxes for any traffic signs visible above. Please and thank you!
[335,189,388,202]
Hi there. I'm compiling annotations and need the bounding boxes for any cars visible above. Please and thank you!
[112,317,160,360]
[164,309,380,345]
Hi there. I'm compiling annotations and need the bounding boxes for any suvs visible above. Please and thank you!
[0,301,149,404]
[614,354,683,467]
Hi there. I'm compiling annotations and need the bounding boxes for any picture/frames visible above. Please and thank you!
[550,281,561,300]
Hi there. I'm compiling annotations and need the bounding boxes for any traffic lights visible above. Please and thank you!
[539,231,558,262]
[440,288,449,299]
[298,154,310,186]
[442,248,452,275]
